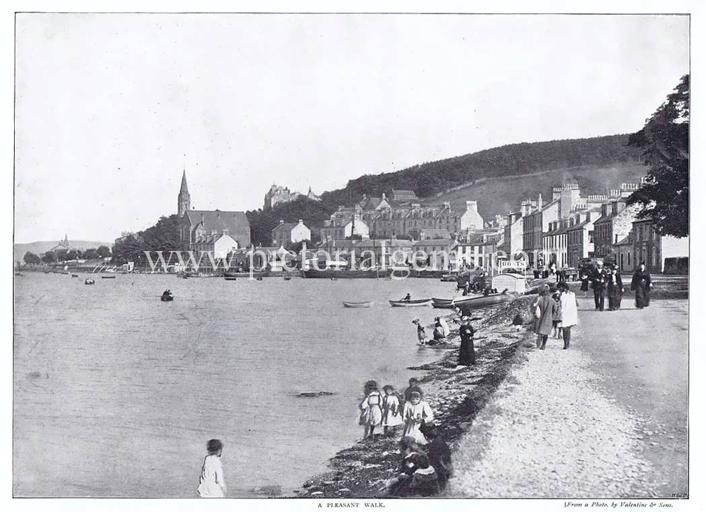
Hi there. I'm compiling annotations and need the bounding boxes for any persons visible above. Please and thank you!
[358,377,454,498]
[413,258,654,366]
[197,437,225,496]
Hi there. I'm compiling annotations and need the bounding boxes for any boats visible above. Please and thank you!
[343,302,374,308]
[440,275,457,280]
[432,294,510,308]
[161,296,173,301]
[284,275,290,280]
[85,279,95,284]
[225,277,236,280]
[389,299,431,307]
